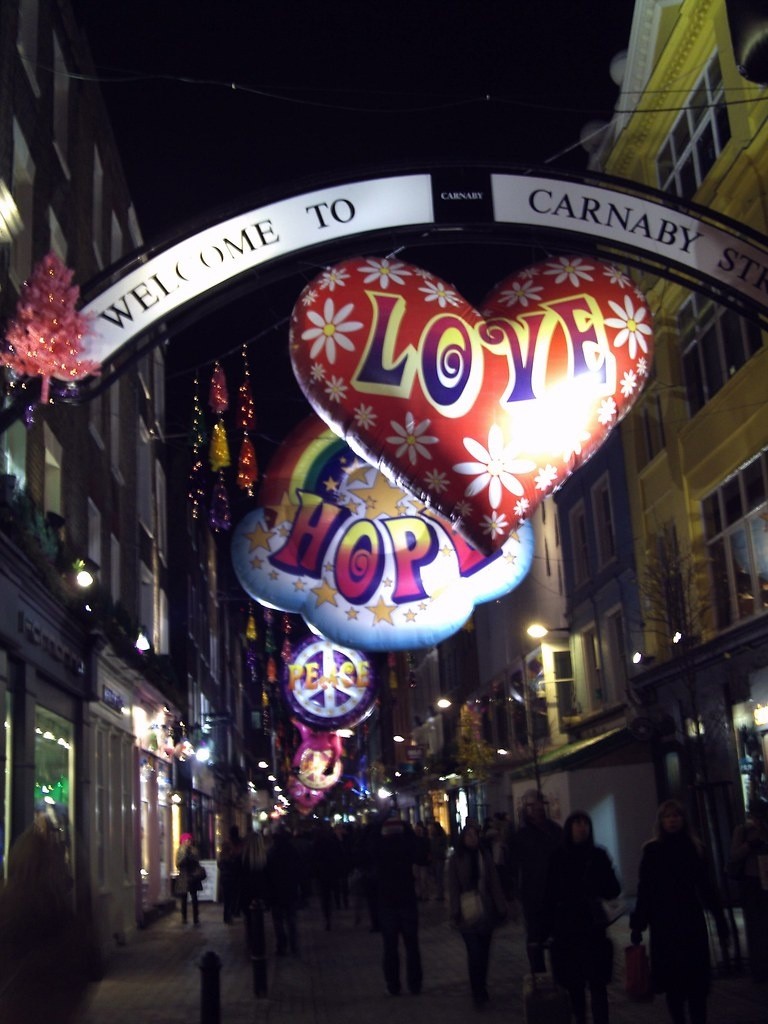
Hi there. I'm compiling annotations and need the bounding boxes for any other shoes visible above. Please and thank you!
[473,987,488,1007]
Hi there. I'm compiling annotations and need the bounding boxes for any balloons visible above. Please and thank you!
[286,716,361,811]
[287,256,656,558]
[231,412,533,651]
[284,636,379,732]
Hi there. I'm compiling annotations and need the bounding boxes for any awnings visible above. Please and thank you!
[508,724,631,782]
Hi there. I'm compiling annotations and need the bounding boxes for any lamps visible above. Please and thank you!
[145,721,216,764]
[436,690,459,709]
[134,624,151,651]
[631,651,651,666]
[73,556,100,590]
[525,623,571,637]
[392,734,412,743]
[673,630,692,647]
[45,510,66,535]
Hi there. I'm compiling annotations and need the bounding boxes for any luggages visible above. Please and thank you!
[522,944,572,1024]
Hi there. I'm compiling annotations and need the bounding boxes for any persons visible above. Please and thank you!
[731,796,768,980]
[216,811,524,958]
[513,789,621,1024]
[364,817,426,995]
[0,828,88,1024]
[629,799,731,1024]
[449,825,510,1007]
[177,833,203,925]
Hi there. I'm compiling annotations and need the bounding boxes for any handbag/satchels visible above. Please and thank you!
[459,891,482,925]
[757,853,768,890]
[721,823,749,908]
[625,942,653,1003]
